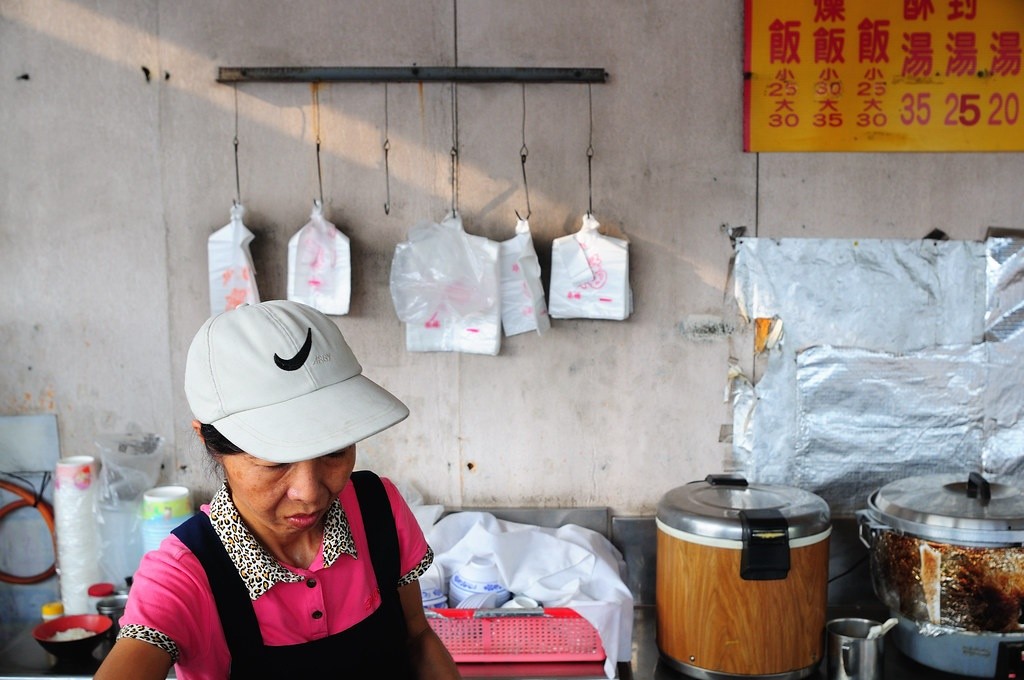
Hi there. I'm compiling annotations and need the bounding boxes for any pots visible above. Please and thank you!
[857,470,1024,678]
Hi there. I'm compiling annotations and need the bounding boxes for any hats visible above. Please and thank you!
[184,299,408,463]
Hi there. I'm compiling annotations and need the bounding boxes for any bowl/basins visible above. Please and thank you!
[50,456,195,615]
[412,549,540,614]
[33,611,114,661]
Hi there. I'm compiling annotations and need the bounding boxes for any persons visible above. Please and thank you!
[92,299,464,680]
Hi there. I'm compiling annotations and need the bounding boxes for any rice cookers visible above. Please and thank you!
[653,468,834,678]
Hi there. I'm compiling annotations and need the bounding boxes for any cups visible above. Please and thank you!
[826,615,887,680]
